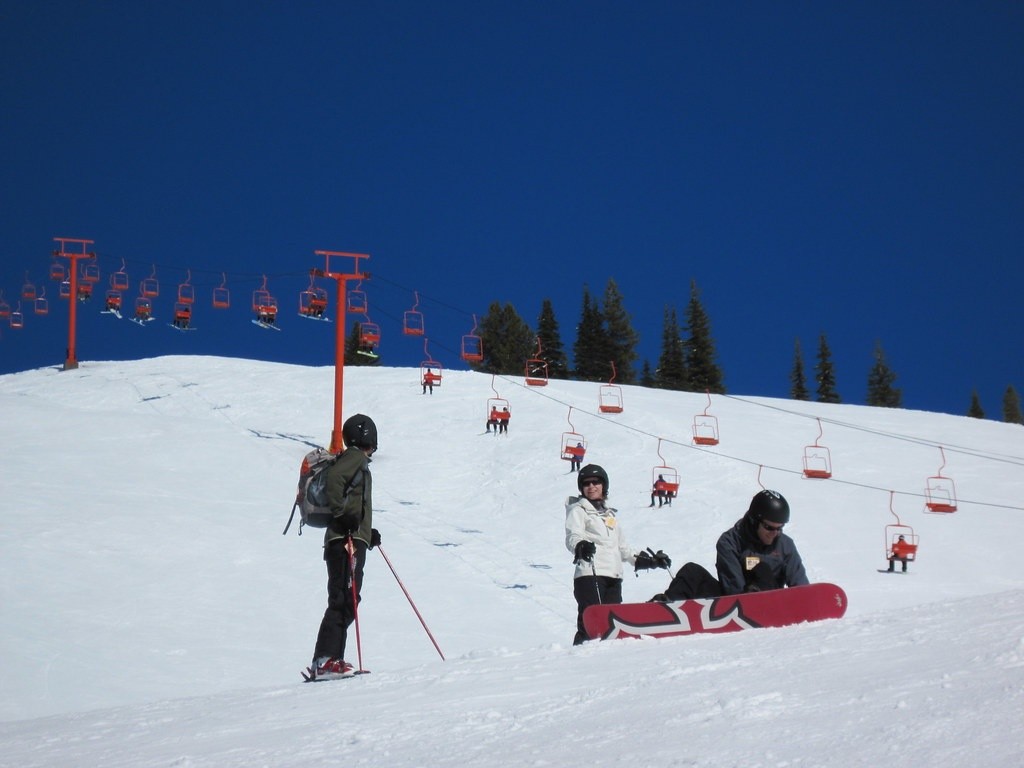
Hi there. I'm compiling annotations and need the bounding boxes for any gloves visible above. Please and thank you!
[334,516,359,535]
[574,540,597,564]
[370,528,382,548]
[651,550,670,570]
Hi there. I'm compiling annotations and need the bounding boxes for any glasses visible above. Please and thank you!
[760,519,783,532]
[582,479,602,487]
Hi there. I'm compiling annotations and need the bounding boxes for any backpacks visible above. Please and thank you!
[297,446,362,528]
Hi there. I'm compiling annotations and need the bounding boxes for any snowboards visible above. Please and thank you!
[581,583,847,640]
[877,569,915,575]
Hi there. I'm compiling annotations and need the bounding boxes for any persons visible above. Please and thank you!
[565,464,671,645]
[486,406,510,435]
[888,535,908,573]
[309,414,382,682]
[78,282,191,327]
[254,292,378,353]
[423,369,434,395]
[649,475,673,508]
[570,442,584,471]
[648,489,810,603]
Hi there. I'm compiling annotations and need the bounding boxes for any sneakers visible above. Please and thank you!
[310,655,354,679]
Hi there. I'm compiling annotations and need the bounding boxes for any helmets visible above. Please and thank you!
[751,491,790,525]
[578,465,608,499]
[342,414,377,450]
